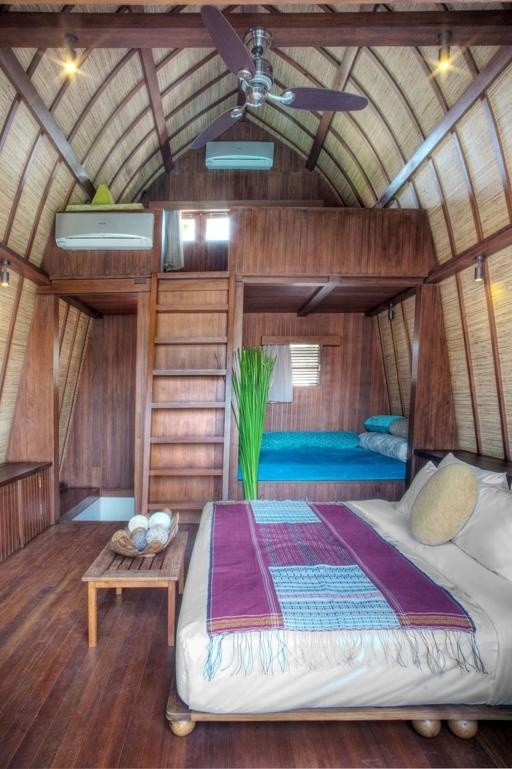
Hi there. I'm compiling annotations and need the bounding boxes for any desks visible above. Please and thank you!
[81,529,189,648]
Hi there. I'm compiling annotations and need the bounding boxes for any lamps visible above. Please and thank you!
[474,255,485,282]
[0,257,11,285]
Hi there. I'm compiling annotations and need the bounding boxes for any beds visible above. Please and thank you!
[164,462,512,740]
[238,431,408,502]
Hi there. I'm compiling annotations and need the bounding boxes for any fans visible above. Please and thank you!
[190,4,368,150]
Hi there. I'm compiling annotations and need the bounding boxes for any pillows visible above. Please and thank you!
[395,453,512,584]
[359,414,411,463]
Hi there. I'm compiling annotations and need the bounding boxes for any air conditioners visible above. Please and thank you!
[205,140,275,170]
[55,213,155,250]
